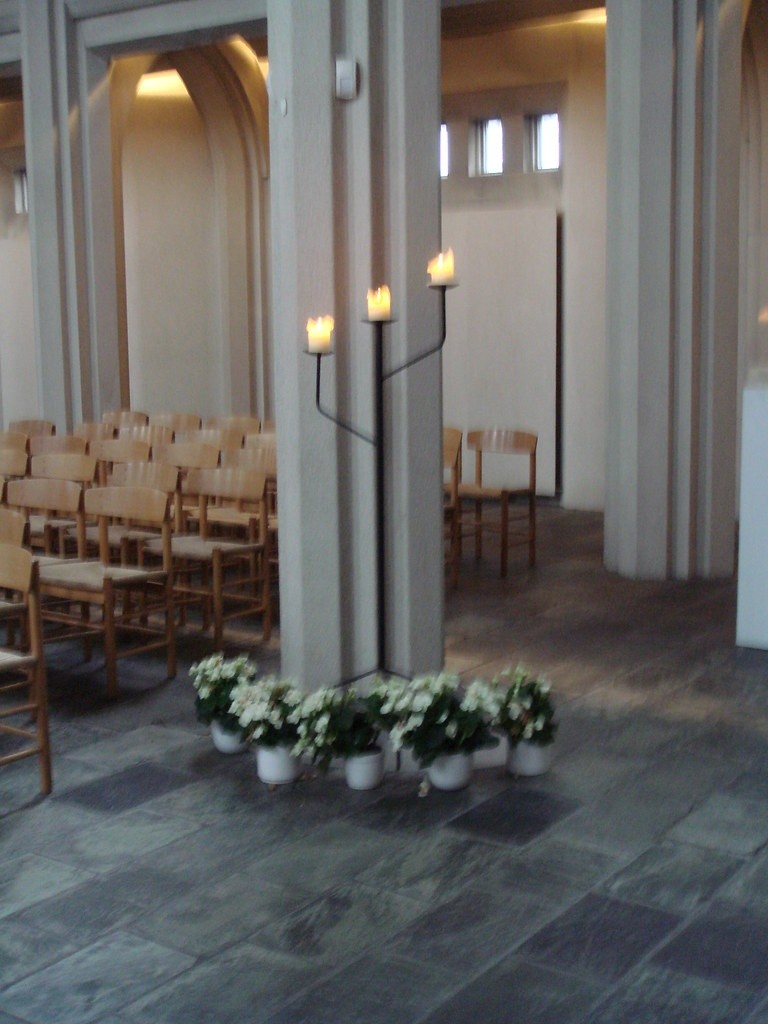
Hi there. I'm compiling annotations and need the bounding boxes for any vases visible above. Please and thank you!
[254,742,303,785]
[341,748,384,790]
[210,718,254,755]
[427,751,476,792]
[507,743,555,776]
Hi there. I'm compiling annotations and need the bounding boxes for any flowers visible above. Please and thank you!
[484,659,561,745]
[189,650,258,729]
[227,675,305,748]
[382,670,500,769]
[295,674,405,771]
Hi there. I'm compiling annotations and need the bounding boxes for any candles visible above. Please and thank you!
[306,314,335,354]
[366,284,391,320]
[427,248,454,285]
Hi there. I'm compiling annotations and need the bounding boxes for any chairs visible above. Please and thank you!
[0,506,38,722]
[117,426,173,444]
[209,433,278,620]
[9,419,56,455]
[261,418,277,434]
[133,465,273,659]
[64,460,183,639]
[74,419,116,443]
[129,442,220,617]
[147,412,202,432]
[27,435,87,454]
[0,544,53,794]
[24,453,107,632]
[444,427,462,554]
[6,477,93,678]
[0,430,28,450]
[102,410,149,428]
[76,437,153,606]
[0,447,30,515]
[170,448,278,636]
[464,428,541,580]
[39,485,177,695]
[170,431,246,613]
[207,415,261,433]
